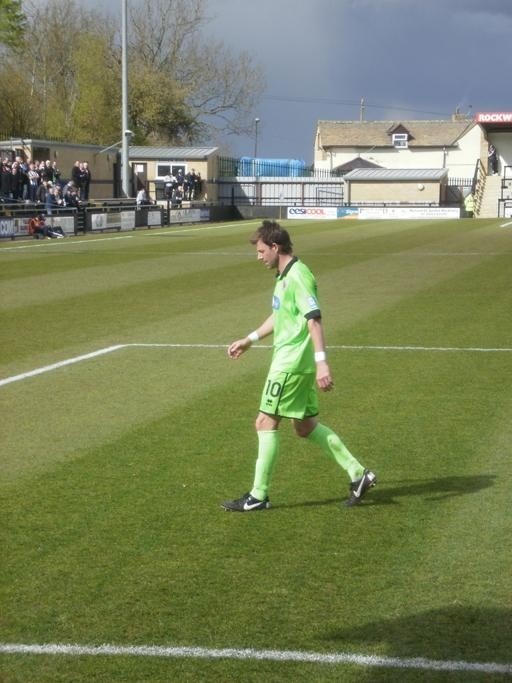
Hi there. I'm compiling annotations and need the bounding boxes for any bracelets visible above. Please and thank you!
[314,351,326,364]
[248,332,260,343]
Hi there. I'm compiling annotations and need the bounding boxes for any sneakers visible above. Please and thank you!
[347,467,377,507]
[220,490,270,512]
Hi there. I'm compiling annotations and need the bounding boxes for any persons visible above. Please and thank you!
[137,168,201,209]
[220,220,377,513]
[488,145,498,176]
[0,156,91,239]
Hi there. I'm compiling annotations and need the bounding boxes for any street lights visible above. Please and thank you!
[254,118,260,158]
